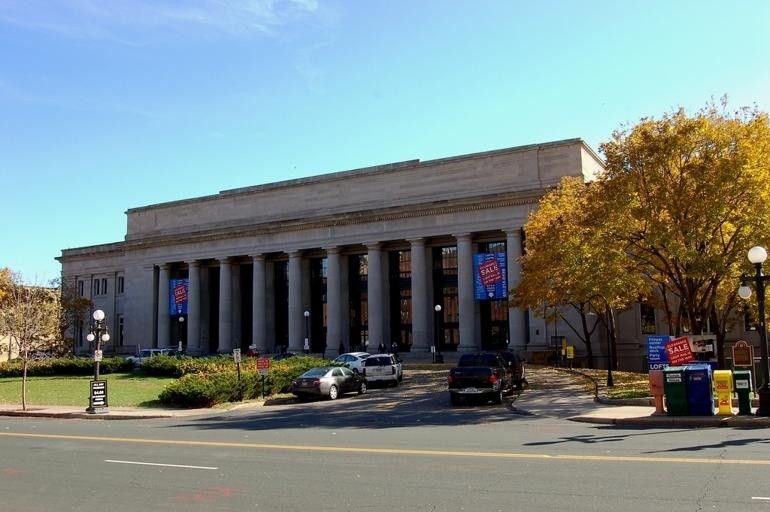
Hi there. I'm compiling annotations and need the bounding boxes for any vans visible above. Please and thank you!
[125,349,174,363]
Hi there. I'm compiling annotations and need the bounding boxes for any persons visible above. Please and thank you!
[390,341,399,354]
[378,342,386,352]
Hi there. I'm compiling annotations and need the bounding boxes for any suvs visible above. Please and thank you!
[492,348,526,389]
[363,352,403,387]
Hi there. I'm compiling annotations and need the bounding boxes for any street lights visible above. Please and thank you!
[85,308,111,409]
[434,305,445,363]
[178,316,184,356]
[304,309,311,355]
[739,245,769,416]
[541,304,560,368]
[586,292,615,388]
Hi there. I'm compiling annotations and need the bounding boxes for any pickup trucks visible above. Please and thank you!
[448,352,512,405]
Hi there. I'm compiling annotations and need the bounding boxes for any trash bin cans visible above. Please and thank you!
[662,365,689,417]
[685,363,715,417]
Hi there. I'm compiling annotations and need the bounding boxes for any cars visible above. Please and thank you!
[291,366,367,399]
[168,348,208,359]
[274,352,298,360]
[332,351,374,367]
[76,353,94,357]
